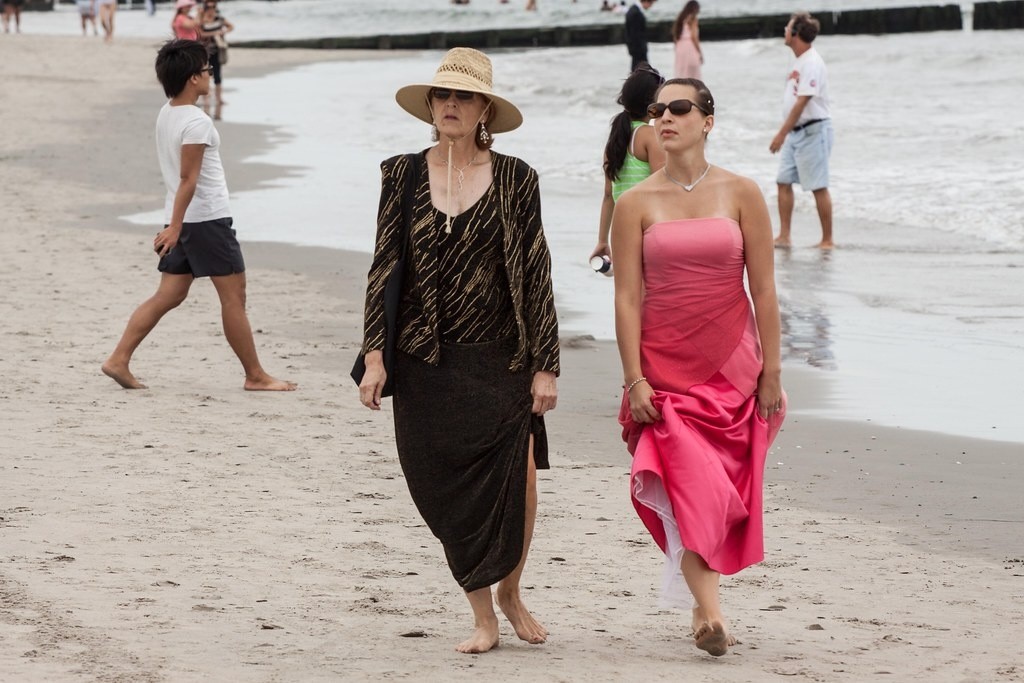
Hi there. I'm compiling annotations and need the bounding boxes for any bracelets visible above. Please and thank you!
[628,378,646,392]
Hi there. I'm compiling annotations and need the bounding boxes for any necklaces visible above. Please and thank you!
[663,162,709,192]
[435,143,481,194]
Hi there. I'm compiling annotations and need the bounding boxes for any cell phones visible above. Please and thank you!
[156,233,171,256]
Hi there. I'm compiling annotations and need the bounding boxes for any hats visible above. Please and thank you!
[174,0,196,9]
[396,47,523,134]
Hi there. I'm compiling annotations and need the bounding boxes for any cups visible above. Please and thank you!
[590,255,614,277]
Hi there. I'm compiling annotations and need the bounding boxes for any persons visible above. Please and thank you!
[586,62,667,262]
[97,0,117,37]
[620,0,656,73]
[3,0,21,32]
[769,14,836,250]
[668,0,703,79]
[611,77,787,656]
[101,38,299,390]
[171,0,234,107]
[526,0,536,11]
[78,0,99,37]
[601,0,629,14]
[349,43,560,655]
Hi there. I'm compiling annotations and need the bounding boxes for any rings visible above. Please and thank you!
[774,408,780,411]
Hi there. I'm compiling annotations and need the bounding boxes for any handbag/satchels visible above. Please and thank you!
[218,47,227,64]
[350,152,422,398]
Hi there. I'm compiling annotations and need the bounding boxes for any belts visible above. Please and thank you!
[792,118,822,132]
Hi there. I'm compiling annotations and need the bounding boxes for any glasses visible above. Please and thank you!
[646,99,710,119]
[192,66,214,76]
[638,67,663,87]
[433,88,475,99]
